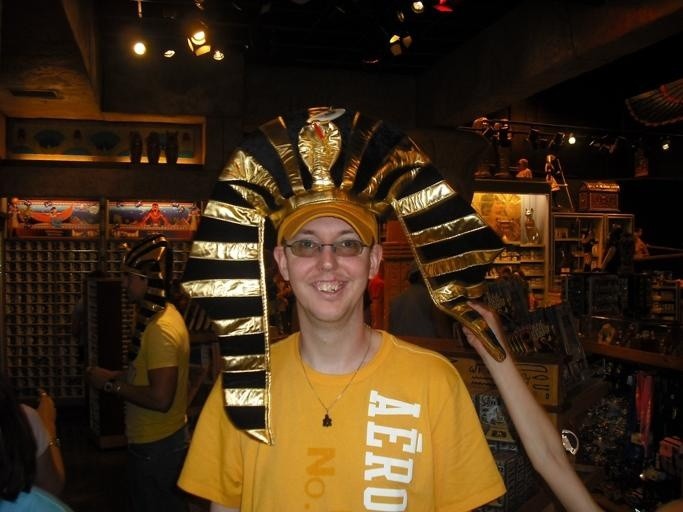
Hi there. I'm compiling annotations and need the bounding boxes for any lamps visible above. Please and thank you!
[476,124,566,153]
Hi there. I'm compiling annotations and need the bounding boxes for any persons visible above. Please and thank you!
[515,154,561,207]
[387,260,450,339]
[368,260,383,328]
[263,249,298,339]
[1,375,74,512]
[463,301,683,512]
[176,216,506,512]
[88,233,194,512]
[600,226,649,275]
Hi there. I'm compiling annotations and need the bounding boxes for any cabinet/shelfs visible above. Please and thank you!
[464,179,635,301]
[2,237,195,411]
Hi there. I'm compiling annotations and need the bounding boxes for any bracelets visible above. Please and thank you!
[112,382,120,392]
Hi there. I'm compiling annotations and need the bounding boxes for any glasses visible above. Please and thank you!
[280,239,372,258]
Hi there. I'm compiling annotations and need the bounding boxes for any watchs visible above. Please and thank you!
[103,378,116,392]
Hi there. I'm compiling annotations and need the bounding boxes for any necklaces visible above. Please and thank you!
[298,322,372,427]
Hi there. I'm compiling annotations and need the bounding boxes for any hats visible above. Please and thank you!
[177,106,509,448]
[119,233,174,363]
